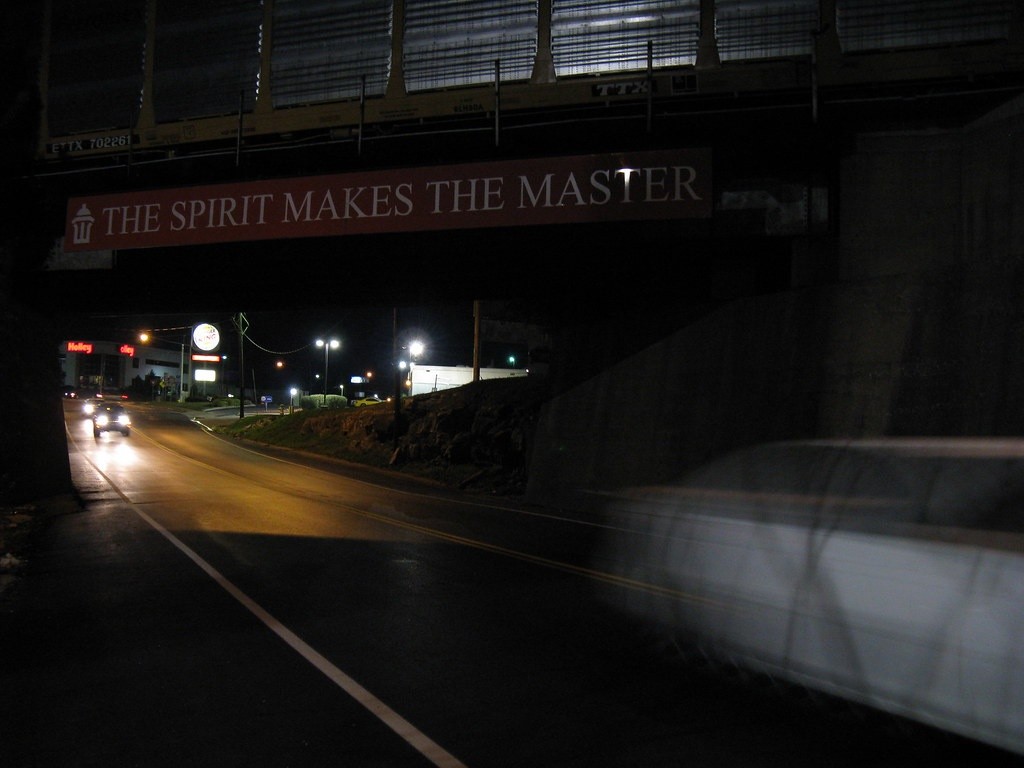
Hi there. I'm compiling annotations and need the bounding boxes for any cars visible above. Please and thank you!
[95,389,129,401]
[62,385,77,399]
[93,402,132,437]
[83,398,106,418]
[351,397,382,409]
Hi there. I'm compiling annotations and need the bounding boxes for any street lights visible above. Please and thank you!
[315,339,341,406]
[398,361,406,409]
